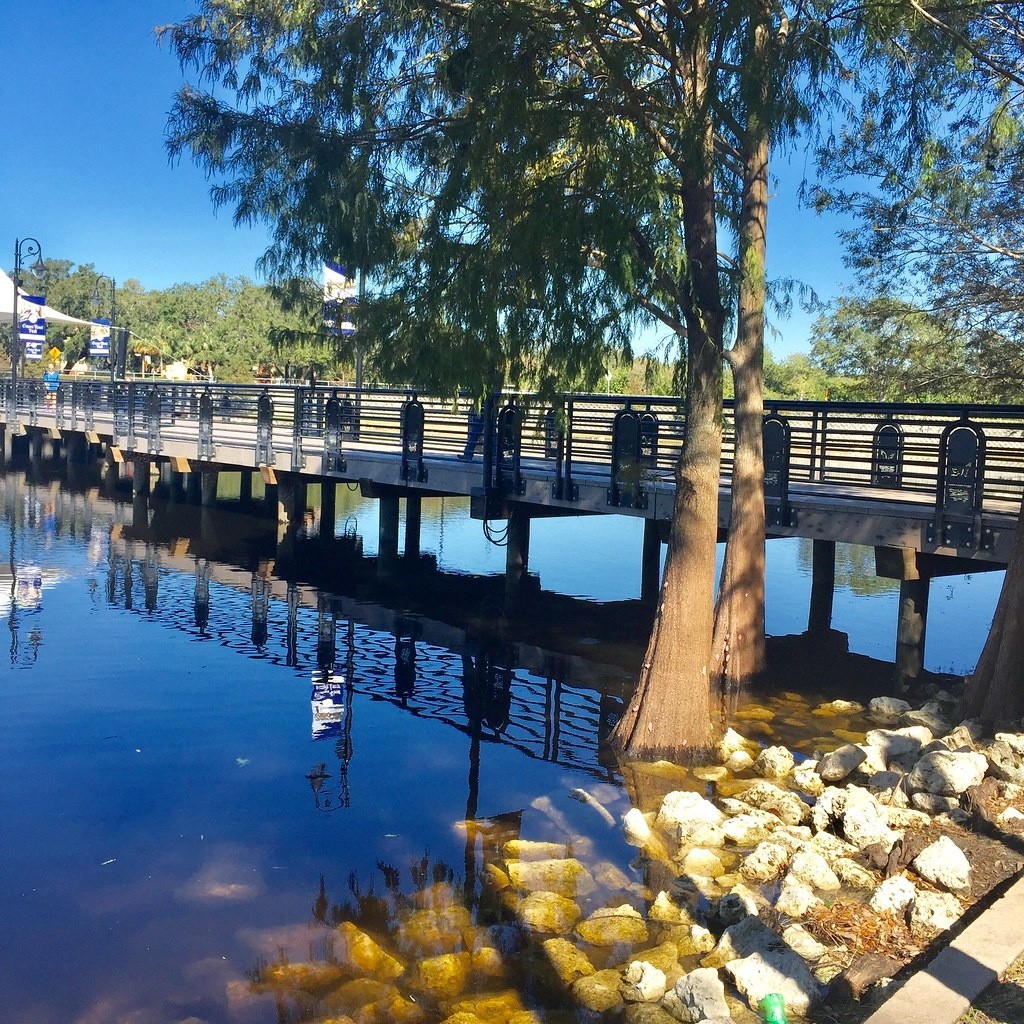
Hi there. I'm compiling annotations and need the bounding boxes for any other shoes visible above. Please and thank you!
[456,454,473,462]
[492,459,497,464]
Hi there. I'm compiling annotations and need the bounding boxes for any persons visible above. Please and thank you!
[43,363,60,413]
[457,364,502,465]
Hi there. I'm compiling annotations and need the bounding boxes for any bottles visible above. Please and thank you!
[765,993,787,1024]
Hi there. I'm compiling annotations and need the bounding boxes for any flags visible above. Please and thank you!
[322,253,357,335]
[19,295,46,360]
[89,318,111,357]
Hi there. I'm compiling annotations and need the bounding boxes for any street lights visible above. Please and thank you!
[8,502,43,670]
[12,236,52,409]
[296,616,358,817]
[90,274,116,381]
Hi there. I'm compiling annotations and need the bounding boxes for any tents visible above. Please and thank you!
[0,268,126,331]
